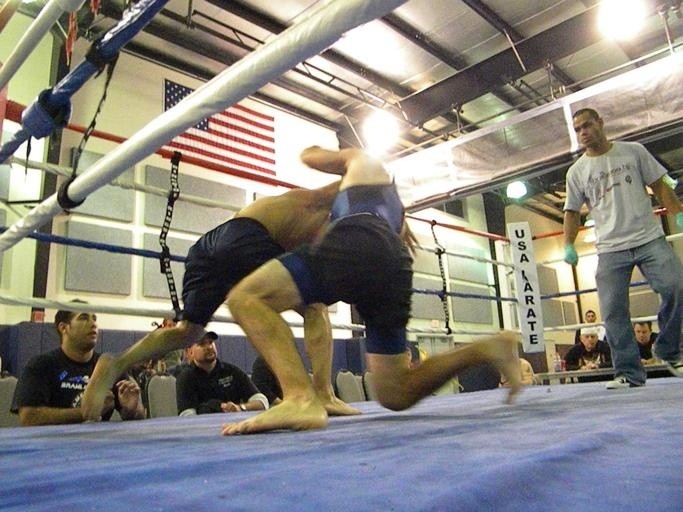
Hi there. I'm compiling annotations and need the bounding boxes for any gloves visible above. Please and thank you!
[564,245,579,266]
[675,212,683,227]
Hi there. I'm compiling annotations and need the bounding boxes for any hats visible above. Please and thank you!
[191,328,219,342]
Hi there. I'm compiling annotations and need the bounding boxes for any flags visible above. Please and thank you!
[161,78,278,189]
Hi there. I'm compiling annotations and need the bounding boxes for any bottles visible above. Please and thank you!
[552,352,561,372]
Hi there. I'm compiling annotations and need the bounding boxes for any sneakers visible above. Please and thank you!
[607,376,638,389]
[665,359,683,377]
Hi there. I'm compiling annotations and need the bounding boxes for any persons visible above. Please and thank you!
[564,328,613,371]
[175,328,270,416]
[79,179,424,421]
[562,108,683,390]
[153,318,185,372]
[219,145,523,437]
[250,354,284,404]
[632,315,658,365]
[9,298,148,426]
[575,310,596,343]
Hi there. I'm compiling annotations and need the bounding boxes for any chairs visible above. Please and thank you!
[147,374,179,417]
[364,370,375,400]
[110,379,143,416]
[336,369,363,403]
[354,372,368,402]
[0,375,28,428]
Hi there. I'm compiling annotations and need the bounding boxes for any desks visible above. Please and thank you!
[536,364,667,383]
